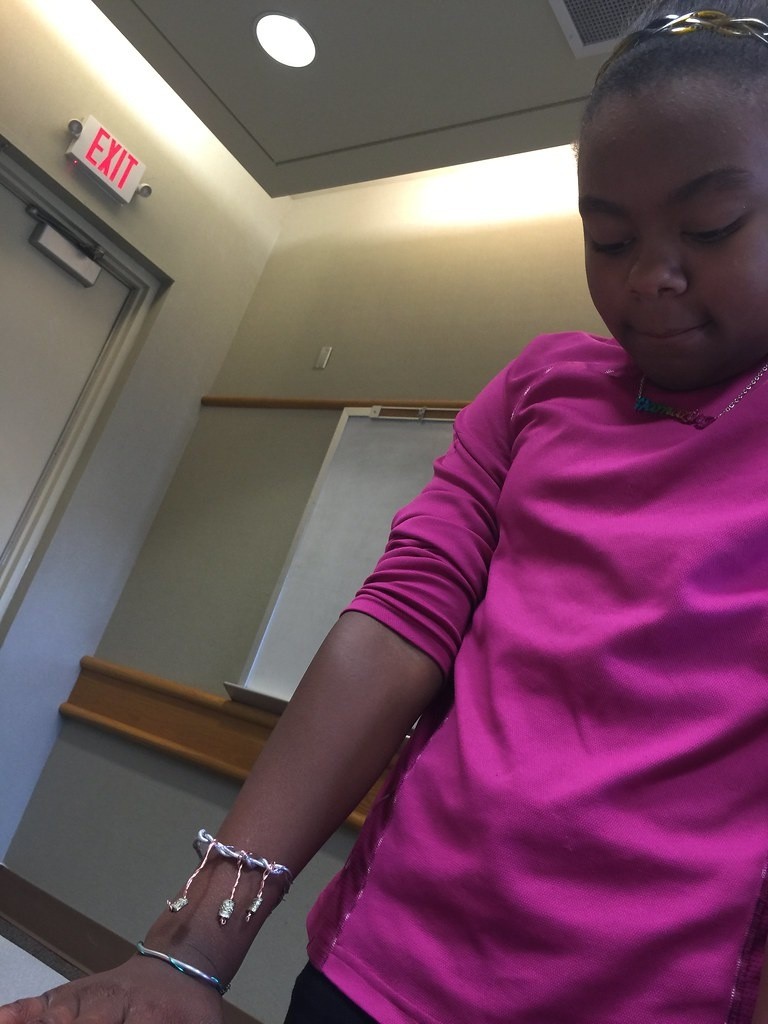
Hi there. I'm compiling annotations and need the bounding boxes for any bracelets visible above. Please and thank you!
[166,829,293,926]
[137,942,229,995]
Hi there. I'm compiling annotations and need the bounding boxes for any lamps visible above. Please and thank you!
[66,112,153,206]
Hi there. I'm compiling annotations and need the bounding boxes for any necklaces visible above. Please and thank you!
[634,366,768,428]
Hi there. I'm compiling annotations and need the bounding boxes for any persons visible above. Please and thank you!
[0,12,768,1024]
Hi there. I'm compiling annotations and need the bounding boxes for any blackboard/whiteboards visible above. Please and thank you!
[225,407,460,710]
